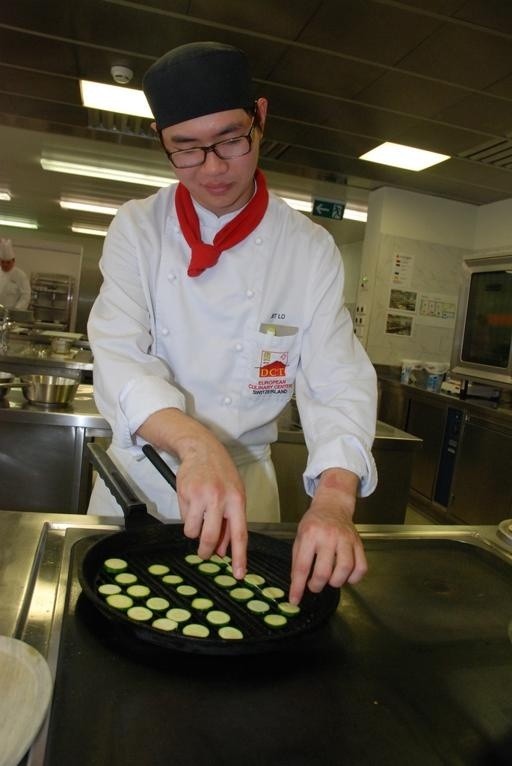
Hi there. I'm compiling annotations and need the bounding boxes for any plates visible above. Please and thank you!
[497,519,512,542]
[2,633,52,766]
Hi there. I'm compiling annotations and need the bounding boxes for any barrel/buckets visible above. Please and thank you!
[399,358,422,387]
[423,360,451,390]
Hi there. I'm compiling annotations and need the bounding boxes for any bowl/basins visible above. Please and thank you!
[51,335,76,355]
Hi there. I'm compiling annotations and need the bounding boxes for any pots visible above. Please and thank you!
[1,374,80,407]
[74,441,344,653]
[1,372,15,398]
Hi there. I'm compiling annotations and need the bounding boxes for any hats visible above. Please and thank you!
[141,40,256,133]
[0,238,15,261]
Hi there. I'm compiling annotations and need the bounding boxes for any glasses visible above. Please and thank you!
[161,117,256,169]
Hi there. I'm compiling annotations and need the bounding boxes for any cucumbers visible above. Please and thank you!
[97,554,301,639]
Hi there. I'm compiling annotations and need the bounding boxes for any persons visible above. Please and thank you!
[86,37,382,610]
[0,236,32,310]
